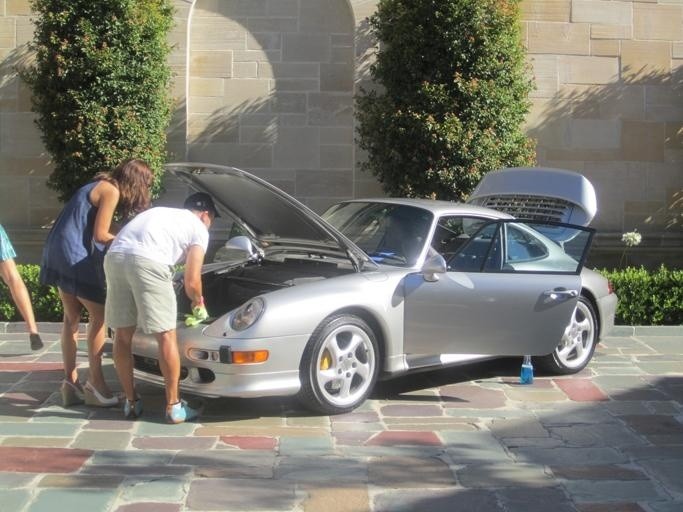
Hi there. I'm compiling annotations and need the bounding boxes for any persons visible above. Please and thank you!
[38,156,155,408]
[102,192,222,424]
[0,223,43,351]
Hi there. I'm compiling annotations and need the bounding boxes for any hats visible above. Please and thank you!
[184,192,221,218]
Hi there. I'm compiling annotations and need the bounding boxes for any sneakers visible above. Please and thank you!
[124,392,143,418]
[166,396,205,424]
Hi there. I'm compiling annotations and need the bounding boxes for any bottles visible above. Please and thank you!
[521,355,534,384]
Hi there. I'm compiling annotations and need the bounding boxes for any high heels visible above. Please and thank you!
[61,379,85,406]
[83,380,120,407]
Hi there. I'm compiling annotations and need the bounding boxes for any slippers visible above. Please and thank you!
[31,333,44,350]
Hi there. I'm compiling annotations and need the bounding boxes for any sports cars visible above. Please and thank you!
[111,162,617,412]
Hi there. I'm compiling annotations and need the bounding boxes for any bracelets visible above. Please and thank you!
[192,296,204,306]
